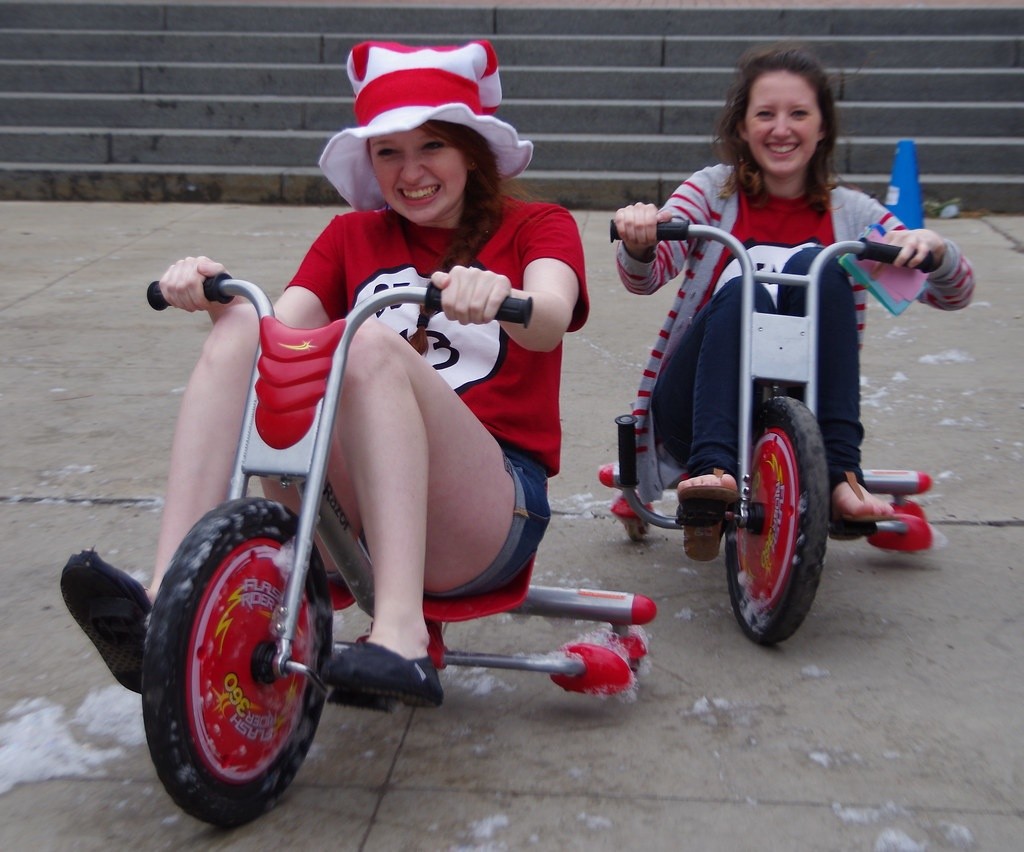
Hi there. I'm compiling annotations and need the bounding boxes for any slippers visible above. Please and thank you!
[826,470,896,541]
[675,467,741,561]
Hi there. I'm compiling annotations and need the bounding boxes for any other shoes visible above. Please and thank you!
[319,642,444,710]
[59,546,154,694]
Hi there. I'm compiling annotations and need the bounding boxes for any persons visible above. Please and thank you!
[617,42,976,565]
[60,41,592,711]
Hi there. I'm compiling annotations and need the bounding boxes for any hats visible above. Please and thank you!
[318,39,533,212]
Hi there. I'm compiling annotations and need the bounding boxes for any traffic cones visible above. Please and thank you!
[882,141,928,230]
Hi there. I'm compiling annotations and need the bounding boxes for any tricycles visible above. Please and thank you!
[137,267,666,830]
[589,214,933,652]
